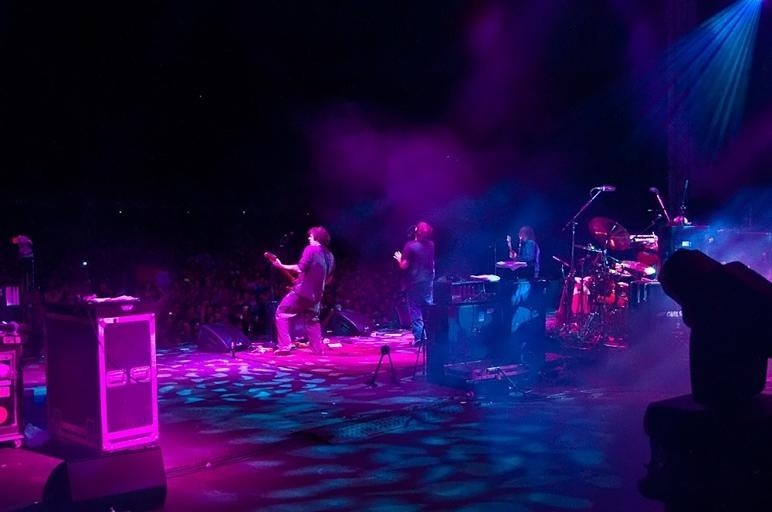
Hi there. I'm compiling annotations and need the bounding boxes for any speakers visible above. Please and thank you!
[422,279,547,390]
[629,280,691,384]
[43,445,168,512]
[196,322,251,354]
[331,307,371,336]
[271,316,309,348]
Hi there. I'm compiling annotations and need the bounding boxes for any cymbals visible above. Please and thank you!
[574,242,608,253]
[588,217,631,252]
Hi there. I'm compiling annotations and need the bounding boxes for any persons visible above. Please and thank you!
[0,257,291,353]
[270,225,335,357]
[507,225,542,280]
[614,244,659,280]
[325,261,403,330]
[393,221,438,346]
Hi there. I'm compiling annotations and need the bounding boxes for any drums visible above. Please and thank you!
[609,268,634,284]
[615,259,657,284]
[571,277,615,317]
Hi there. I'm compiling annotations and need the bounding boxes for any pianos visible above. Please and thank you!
[627,233,659,246]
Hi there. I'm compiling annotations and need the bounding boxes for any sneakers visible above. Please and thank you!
[412,338,427,347]
[274,349,290,355]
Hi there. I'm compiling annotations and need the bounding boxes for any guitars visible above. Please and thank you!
[262,251,300,292]
[506,234,515,262]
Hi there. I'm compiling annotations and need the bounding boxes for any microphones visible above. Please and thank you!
[596,185,616,192]
[552,255,570,267]
[649,187,659,194]
